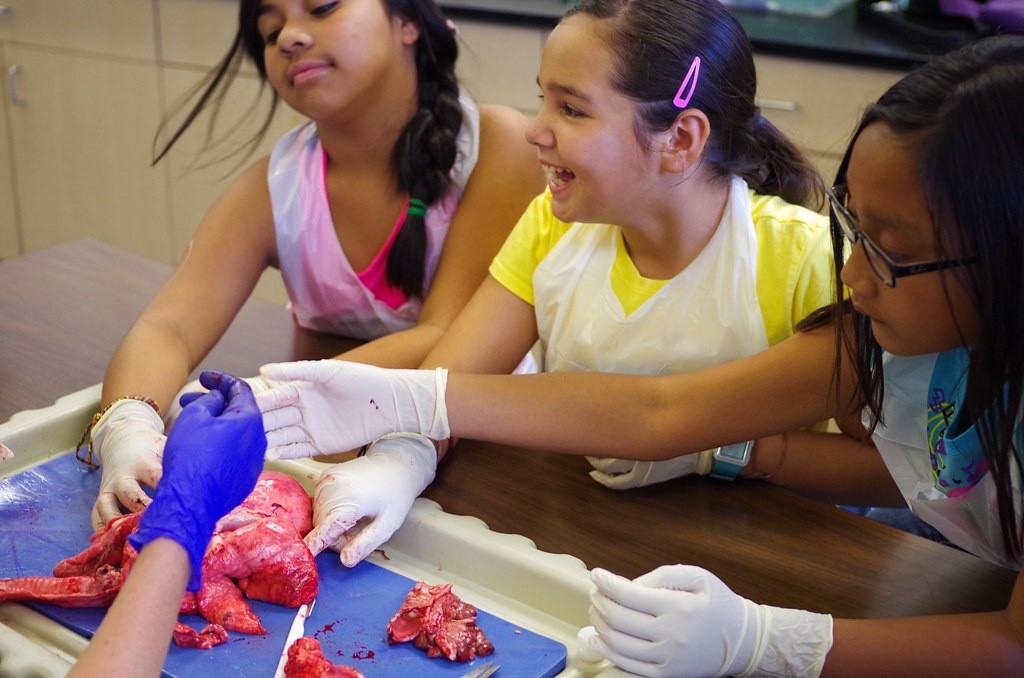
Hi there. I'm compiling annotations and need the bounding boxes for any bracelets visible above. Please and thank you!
[75,394,162,468]
[767,430,788,481]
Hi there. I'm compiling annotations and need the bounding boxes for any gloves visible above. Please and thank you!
[127,371,267,593]
[302,432,437,568]
[585,449,713,489]
[255,359,451,461]
[91,399,169,532]
[163,375,270,437]
[588,564,833,678]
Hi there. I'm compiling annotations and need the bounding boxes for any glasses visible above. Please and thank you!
[825,184,975,289]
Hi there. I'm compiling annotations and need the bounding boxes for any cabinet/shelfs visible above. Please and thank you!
[450,16,914,218]
[0,0,178,280]
[153,0,310,306]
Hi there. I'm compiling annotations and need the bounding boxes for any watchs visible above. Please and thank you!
[712,436,755,481]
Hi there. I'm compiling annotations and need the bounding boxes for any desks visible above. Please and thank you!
[1,236,1017,678]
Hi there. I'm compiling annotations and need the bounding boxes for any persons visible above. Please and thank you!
[301,0,892,568]
[0,371,268,678]
[257,35,1024,678]
[73,0,546,578]
